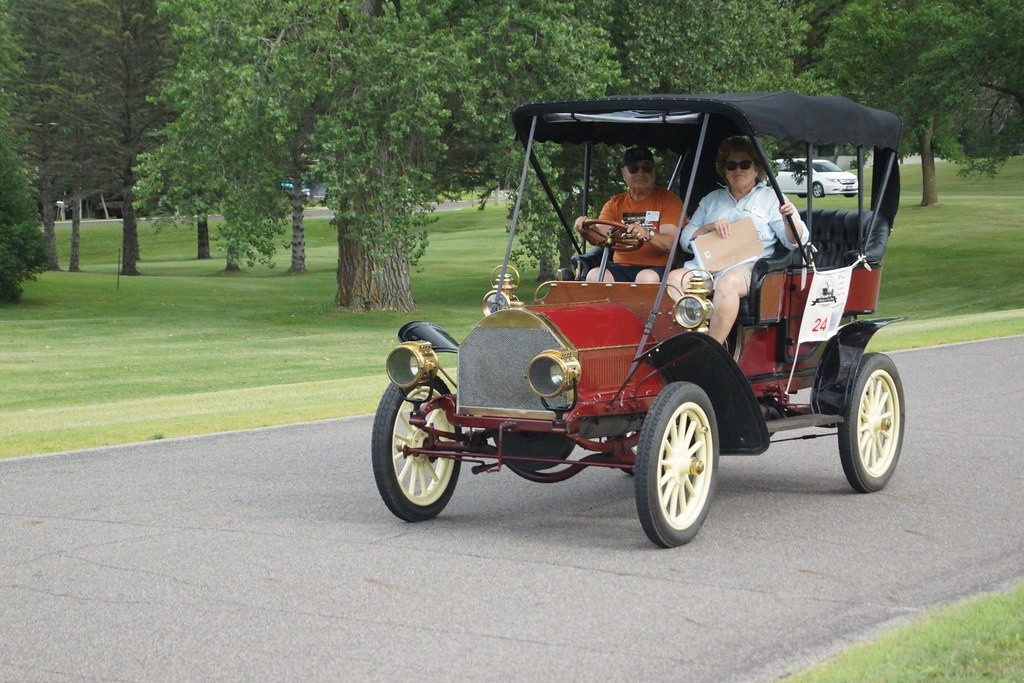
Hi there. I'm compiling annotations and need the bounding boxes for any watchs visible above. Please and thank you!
[644,229,654,242]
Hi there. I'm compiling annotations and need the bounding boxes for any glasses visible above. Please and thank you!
[724,160,755,170]
[625,165,655,174]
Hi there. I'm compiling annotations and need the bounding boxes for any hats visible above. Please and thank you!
[623,147,654,167]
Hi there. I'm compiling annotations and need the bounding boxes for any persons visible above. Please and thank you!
[667,138,810,344]
[575,147,687,284]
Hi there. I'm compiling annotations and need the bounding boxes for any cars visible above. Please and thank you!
[371,90,906,550]
[770,158,857,200]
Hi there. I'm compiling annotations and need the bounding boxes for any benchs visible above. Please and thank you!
[734,208,886,324]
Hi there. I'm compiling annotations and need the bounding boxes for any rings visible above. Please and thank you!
[719,226,722,227]
[638,232,641,235]
